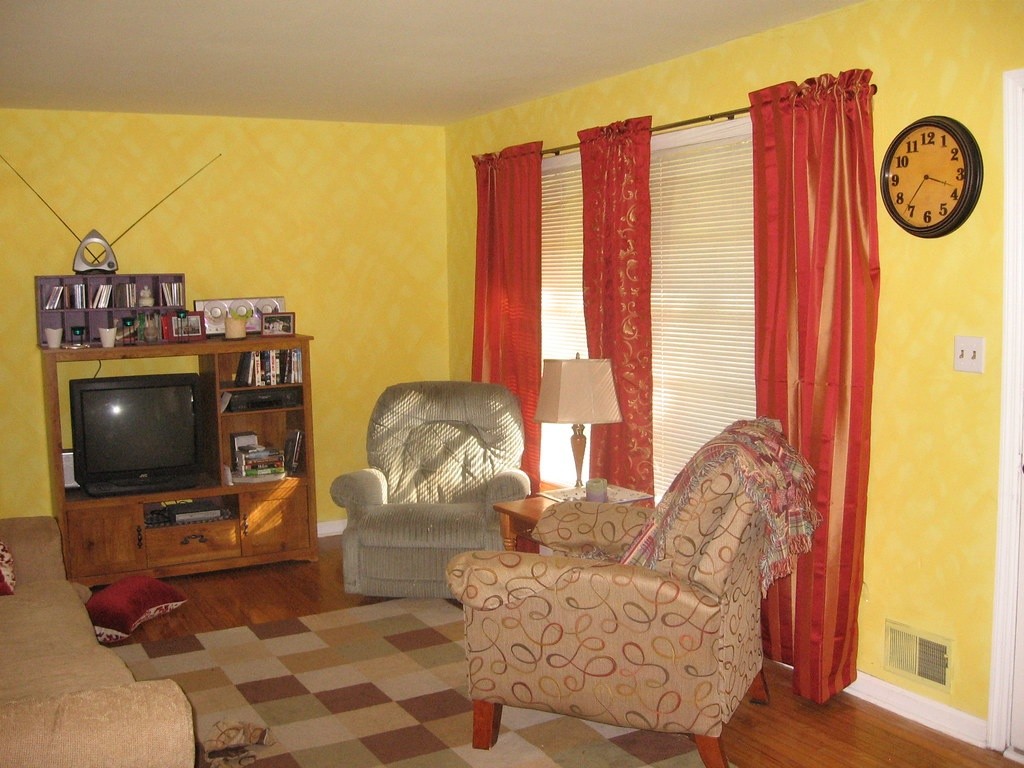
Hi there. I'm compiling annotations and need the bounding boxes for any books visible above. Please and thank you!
[219,350,304,483]
[40,282,183,309]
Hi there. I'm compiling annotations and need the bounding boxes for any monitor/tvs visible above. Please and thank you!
[69,373,205,496]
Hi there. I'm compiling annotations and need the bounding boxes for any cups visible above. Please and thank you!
[45,328,63,348]
[98,327,117,348]
[143,327,158,342]
[224,317,247,339]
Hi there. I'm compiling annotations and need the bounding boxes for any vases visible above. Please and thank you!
[98,327,117,347]
[45,328,63,348]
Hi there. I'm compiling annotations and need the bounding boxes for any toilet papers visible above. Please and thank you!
[585,478,608,502]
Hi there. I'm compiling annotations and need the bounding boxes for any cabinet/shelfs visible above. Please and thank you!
[41,332,319,589]
[35,273,186,349]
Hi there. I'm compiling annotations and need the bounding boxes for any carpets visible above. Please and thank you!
[108,598,738,768]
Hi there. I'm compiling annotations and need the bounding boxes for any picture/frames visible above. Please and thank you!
[166,311,207,343]
[261,312,295,337]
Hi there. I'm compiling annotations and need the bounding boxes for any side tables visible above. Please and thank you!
[493,484,654,554]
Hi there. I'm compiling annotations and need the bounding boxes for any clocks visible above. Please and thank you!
[880,115,985,239]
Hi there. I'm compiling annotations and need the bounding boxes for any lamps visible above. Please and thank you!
[534,352,622,487]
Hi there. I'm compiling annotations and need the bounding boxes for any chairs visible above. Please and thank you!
[446,417,822,768]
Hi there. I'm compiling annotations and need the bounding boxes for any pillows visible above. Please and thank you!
[86,575,188,642]
[0,541,16,595]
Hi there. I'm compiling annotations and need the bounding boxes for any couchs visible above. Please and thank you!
[0,516,196,768]
[330,381,531,599]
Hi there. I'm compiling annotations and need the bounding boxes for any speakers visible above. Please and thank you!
[194,296,285,335]
